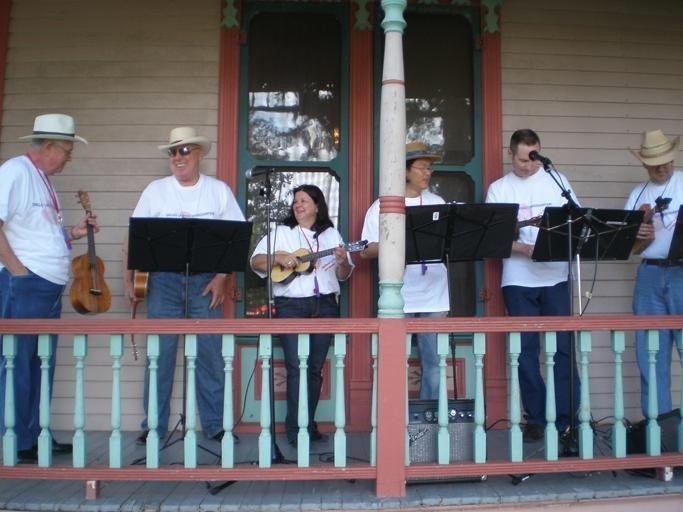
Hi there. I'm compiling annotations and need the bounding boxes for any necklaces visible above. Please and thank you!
[405,192,428,208]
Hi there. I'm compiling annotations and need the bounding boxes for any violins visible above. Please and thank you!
[131,271,149,359]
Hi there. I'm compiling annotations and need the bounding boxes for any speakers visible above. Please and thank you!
[626,408,681,479]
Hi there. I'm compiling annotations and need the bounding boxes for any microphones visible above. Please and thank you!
[245,167,278,179]
[528,150,552,165]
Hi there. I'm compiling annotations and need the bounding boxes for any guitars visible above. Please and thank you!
[272,237,369,281]
[629,196,672,254]
[516,213,543,234]
[72,190,110,313]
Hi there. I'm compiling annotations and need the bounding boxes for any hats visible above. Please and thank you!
[628,128,682,167]
[157,126,211,156]
[405,143,442,163]
[18,113,87,145]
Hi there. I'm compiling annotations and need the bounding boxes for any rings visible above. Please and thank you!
[288,260,292,265]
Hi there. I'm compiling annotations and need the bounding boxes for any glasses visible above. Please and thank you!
[409,164,432,171]
[51,142,73,157]
[167,145,200,157]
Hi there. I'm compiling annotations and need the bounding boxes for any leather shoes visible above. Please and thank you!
[16,438,72,464]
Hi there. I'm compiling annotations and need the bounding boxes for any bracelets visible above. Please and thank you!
[218,273,231,279]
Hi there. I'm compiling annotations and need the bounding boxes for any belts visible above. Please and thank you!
[639,257,683,268]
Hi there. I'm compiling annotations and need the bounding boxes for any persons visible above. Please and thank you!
[125,126,248,443]
[250,184,356,450]
[484,129,583,441]
[0,113,100,459]
[360,142,451,402]
[624,130,683,418]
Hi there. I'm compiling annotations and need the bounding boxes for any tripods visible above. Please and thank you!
[510,255,618,485]
[203,208,359,496]
[130,264,220,466]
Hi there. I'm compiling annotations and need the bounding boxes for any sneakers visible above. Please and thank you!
[523,425,545,443]
[213,431,240,444]
[290,430,329,449]
[135,432,165,446]
